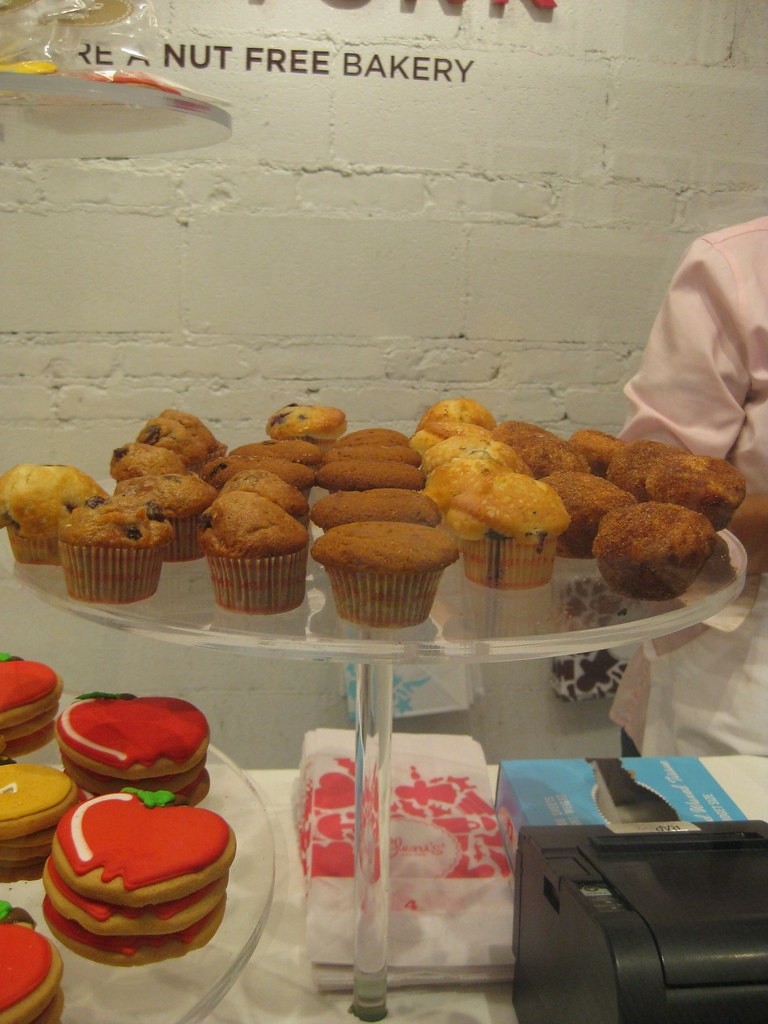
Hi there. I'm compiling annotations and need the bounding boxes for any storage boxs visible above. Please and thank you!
[493,755,768,871]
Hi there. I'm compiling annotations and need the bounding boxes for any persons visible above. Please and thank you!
[609,217,768,759]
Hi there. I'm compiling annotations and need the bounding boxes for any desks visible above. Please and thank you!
[0,765,523,1024]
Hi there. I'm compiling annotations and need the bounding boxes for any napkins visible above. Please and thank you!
[295,728,517,992]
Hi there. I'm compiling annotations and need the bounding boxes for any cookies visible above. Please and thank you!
[0,652,238,1024]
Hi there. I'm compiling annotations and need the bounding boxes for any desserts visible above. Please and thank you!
[0,399,746,629]
